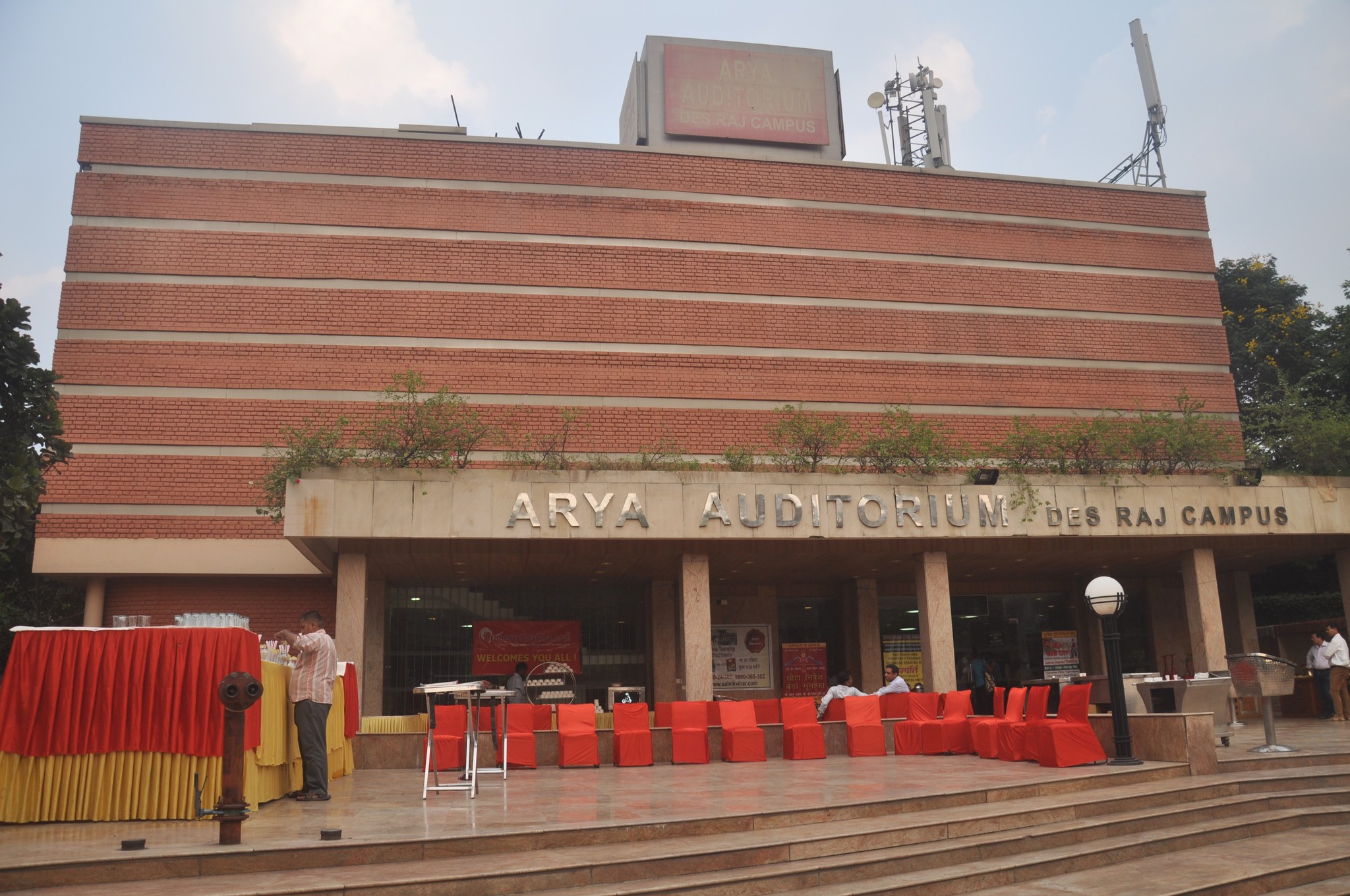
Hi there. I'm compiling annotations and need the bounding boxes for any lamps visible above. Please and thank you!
[1237,468,1263,487]
[974,469,1000,485]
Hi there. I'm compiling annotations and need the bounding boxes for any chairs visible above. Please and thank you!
[1024,685,1076,763]
[719,701,767,762]
[558,703,600,769]
[780,697,827,760]
[844,695,889,757]
[996,686,1051,761]
[920,690,972,754]
[671,701,710,764]
[894,692,939,754]
[967,687,1006,756]
[423,705,466,772]
[612,703,654,767]
[497,704,537,771]
[1033,683,1108,767]
[975,687,1027,759]
[455,686,982,734]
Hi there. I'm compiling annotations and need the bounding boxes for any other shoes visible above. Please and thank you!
[1326,717,1345,721]
[1318,715,1328,719]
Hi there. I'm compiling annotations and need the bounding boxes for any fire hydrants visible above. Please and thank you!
[193,670,265,846]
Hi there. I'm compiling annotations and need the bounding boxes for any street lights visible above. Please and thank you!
[1082,576,1144,765]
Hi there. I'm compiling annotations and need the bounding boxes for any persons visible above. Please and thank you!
[1322,621,1350,722]
[274,607,333,803]
[1306,628,1337,719]
[981,650,1001,715]
[871,664,910,698]
[816,671,870,722]
[968,649,998,716]
[506,662,529,705]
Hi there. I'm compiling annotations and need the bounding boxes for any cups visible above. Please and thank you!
[1165,674,1169,680]
[1174,675,1178,681]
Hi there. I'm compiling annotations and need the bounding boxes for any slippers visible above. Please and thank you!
[295,791,332,801]
[288,790,304,798]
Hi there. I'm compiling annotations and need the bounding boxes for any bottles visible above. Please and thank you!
[111,614,152,628]
[262,647,296,669]
[173,612,249,630]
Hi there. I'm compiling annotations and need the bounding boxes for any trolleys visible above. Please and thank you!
[1132,676,1234,747]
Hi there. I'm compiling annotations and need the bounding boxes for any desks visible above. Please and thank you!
[1280,678,1324,719]
